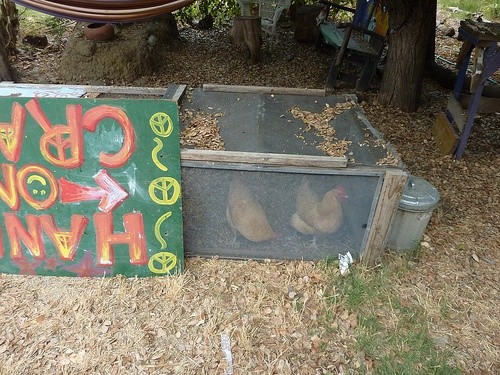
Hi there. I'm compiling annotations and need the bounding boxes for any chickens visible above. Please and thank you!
[290,175,349,250]
[226,173,280,248]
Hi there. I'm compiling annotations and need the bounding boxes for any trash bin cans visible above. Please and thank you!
[386,175,442,250]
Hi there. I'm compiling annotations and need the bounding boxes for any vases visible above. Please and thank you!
[83,23,114,42]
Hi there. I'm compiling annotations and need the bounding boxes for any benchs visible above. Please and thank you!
[310,0,393,93]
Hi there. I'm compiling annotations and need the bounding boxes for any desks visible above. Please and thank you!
[444,20,500,162]
